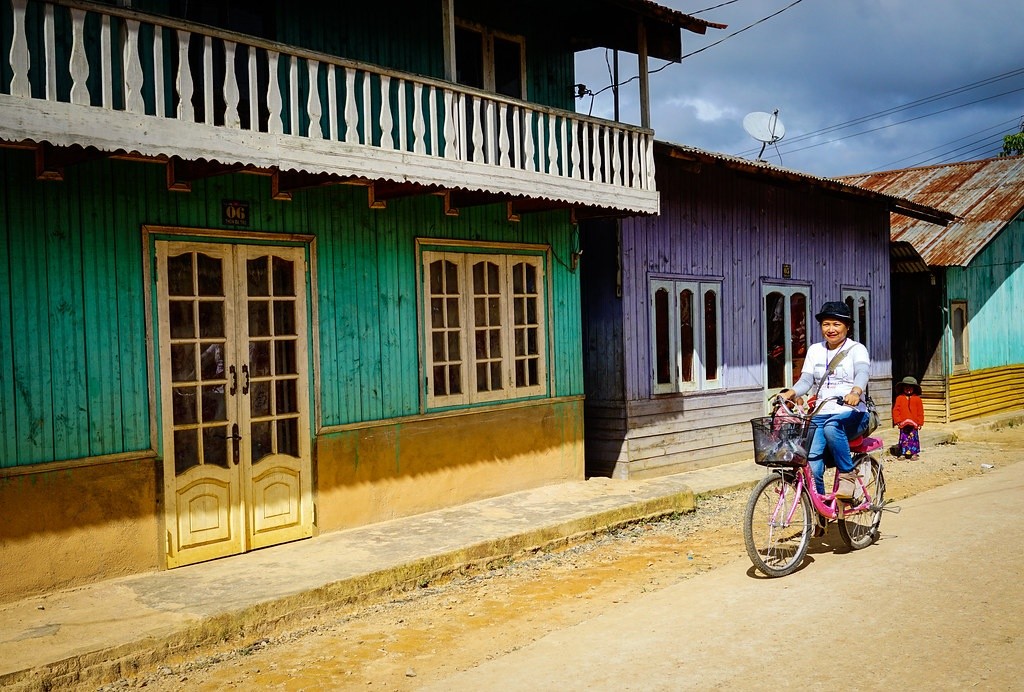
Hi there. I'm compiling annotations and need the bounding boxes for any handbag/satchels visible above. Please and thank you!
[863,396,880,437]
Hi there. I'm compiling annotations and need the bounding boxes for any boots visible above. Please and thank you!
[835,468,856,498]
[813,512,828,537]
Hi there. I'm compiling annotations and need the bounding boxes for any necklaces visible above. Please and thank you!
[824,337,847,388]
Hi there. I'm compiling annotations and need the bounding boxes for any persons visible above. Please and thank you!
[892,377,924,460]
[770,389,804,440]
[778,301,871,539]
[180,330,256,449]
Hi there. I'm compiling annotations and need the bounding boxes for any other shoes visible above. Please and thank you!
[911,454,918,461]
[898,455,906,461]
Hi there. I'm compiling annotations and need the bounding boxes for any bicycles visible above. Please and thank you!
[742,394,904,578]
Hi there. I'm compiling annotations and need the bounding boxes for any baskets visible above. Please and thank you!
[750,416,818,466]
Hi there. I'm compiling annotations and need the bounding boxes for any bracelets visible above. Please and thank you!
[851,391,861,396]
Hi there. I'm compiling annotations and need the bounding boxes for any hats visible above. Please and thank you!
[815,301,855,323]
[895,376,922,395]
